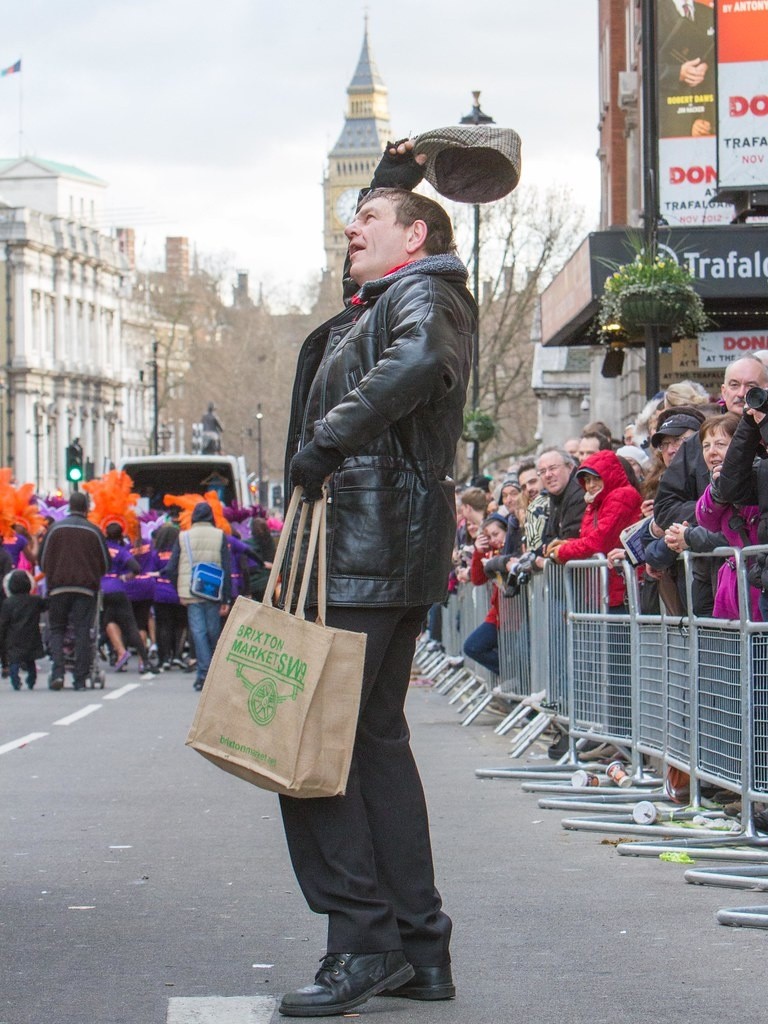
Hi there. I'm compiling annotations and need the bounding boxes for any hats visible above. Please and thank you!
[575,467,600,477]
[652,414,702,447]
[615,445,652,476]
[412,124,521,205]
[501,471,522,492]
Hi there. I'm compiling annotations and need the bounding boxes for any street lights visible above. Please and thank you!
[256,403,263,514]
[458,91,497,488]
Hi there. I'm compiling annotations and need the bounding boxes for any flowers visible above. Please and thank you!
[462,408,496,443]
[586,224,720,347]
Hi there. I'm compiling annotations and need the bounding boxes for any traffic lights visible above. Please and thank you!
[67,444,83,482]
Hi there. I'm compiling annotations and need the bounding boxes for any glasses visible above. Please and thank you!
[536,463,567,477]
[657,435,689,452]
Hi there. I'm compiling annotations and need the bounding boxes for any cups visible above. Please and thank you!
[605,761,632,788]
[571,769,601,787]
[632,800,663,825]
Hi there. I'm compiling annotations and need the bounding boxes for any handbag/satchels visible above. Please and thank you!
[185,478,368,798]
[190,562,225,604]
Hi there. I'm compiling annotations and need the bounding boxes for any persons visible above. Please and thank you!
[240,518,283,602]
[37,492,113,691]
[426,444,586,719]
[548,350,768,832]
[100,522,156,674]
[124,525,190,674]
[277,135,478,1016]
[166,502,232,691]
[656,0,715,136]
[201,400,224,455]
[0,524,48,692]
[226,523,273,599]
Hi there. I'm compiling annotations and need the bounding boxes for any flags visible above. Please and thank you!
[0,58,20,79]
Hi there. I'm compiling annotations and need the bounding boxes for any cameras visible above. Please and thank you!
[746,387,768,415]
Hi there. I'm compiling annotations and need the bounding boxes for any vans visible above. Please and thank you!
[118,454,247,521]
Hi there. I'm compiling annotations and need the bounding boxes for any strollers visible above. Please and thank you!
[41,588,106,690]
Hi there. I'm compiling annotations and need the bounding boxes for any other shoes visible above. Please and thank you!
[0,638,211,692]
[547,716,768,832]
[375,961,457,1000]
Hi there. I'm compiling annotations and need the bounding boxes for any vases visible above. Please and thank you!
[619,292,687,342]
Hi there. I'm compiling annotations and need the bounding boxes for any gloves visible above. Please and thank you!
[291,439,348,501]
[484,554,507,580]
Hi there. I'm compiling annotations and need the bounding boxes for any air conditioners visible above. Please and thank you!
[617,69,639,111]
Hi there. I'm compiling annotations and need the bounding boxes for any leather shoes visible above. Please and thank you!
[278,948,416,1016]
